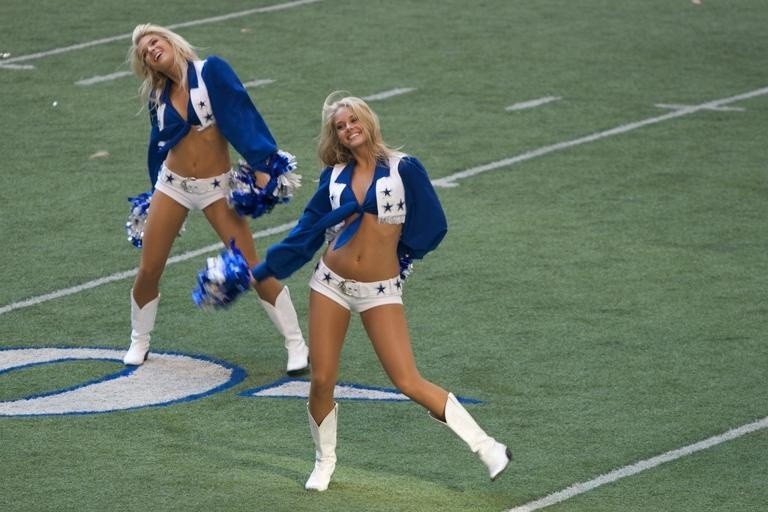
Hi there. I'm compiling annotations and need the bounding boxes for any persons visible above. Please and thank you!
[123,22,308,376]
[248,90,513,493]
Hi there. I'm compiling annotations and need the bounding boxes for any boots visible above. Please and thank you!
[258,285,310,375]
[123,286,161,366]
[425,389,513,482]
[303,400,339,492]
